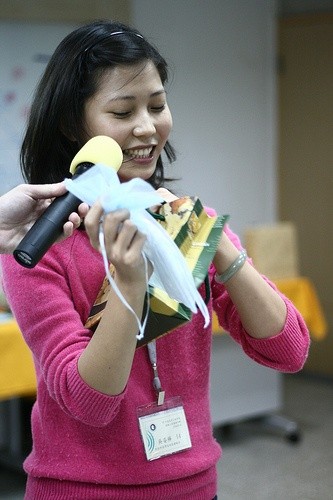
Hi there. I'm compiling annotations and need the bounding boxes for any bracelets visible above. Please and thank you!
[214,252,246,284]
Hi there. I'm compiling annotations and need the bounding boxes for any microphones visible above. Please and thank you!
[14,135,123,268]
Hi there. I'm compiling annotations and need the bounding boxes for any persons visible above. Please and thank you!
[0,23,311,500]
[0,178,90,254]
